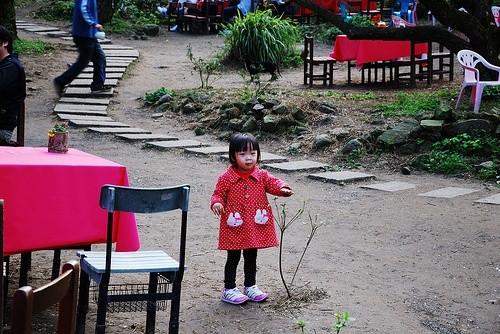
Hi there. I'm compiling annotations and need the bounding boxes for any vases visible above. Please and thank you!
[48,132,69,153]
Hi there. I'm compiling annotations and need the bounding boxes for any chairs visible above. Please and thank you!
[76,184,190,334]
[168,0,262,35]
[361,43,453,84]
[0,101,25,147]
[0,199,80,334]
[338,0,419,25]
[454,50,500,114]
[304,35,338,87]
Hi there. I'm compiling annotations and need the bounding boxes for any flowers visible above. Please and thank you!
[49,121,69,134]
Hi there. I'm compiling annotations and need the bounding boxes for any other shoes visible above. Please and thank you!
[157,7,165,14]
[170,25,178,32]
[54,77,66,97]
[91,86,112,93]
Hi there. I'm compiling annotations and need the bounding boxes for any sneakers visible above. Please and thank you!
[219,287,249,304]
[244,285,269,302]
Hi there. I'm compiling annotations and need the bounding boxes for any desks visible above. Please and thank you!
[329,34,428,86]
[0,145,140,288]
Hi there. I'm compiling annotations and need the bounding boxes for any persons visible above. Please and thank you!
[0,26,25,144]
[157,0,298,32]
[53,0,112,98]
[210,133,295,304]
